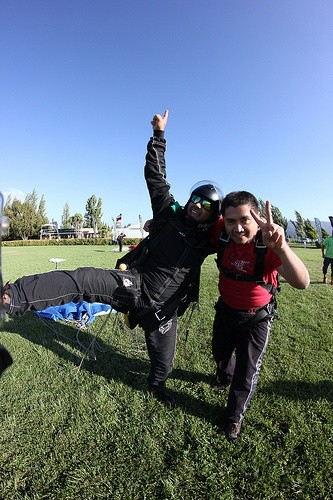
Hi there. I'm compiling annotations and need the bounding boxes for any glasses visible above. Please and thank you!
[190,195,212,212]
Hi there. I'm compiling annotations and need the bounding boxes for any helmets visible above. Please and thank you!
[189,185,223,217]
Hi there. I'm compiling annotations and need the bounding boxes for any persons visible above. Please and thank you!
[0,109,311,442]
[321,230,333,286]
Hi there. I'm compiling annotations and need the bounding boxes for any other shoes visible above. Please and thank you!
[0,277,12,320]
[225,419,242,438]
[140,380,159,394]
[330,280,333,285]
[323,277,327,283]
[214,377,225,390]
